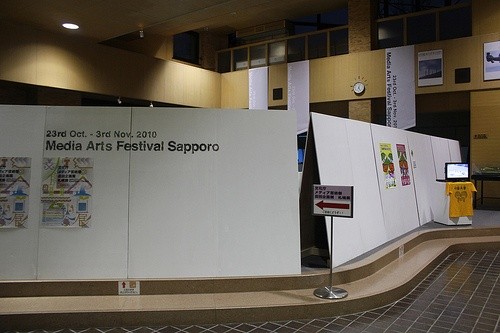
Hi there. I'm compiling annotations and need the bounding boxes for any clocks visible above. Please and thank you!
[353,82,364,93]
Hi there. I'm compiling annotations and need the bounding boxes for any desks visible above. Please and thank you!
[471,173,500,209]
[432,180,477,225]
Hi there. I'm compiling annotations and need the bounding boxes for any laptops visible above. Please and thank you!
[441,162,471,182]
